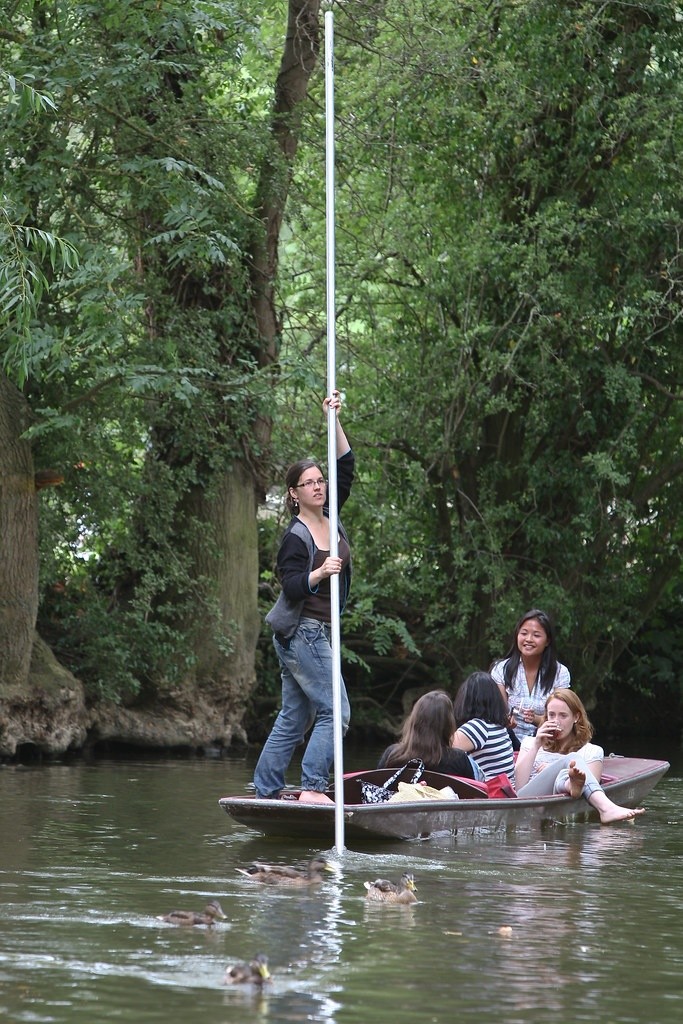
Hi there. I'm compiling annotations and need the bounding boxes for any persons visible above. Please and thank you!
[377,691,486,782]
[452,672,516,790]
[254,391,354,803]
[490,610,570,751]
[515,689,645,824]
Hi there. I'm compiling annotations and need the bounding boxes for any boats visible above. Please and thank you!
[217,754,672,842]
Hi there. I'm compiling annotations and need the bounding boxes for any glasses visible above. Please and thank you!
[294,478,328,487]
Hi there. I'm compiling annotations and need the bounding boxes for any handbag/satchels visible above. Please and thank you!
[355,757,459,804]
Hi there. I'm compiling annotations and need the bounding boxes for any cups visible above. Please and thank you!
[546,722,563,742]
[518,697,534,718]
